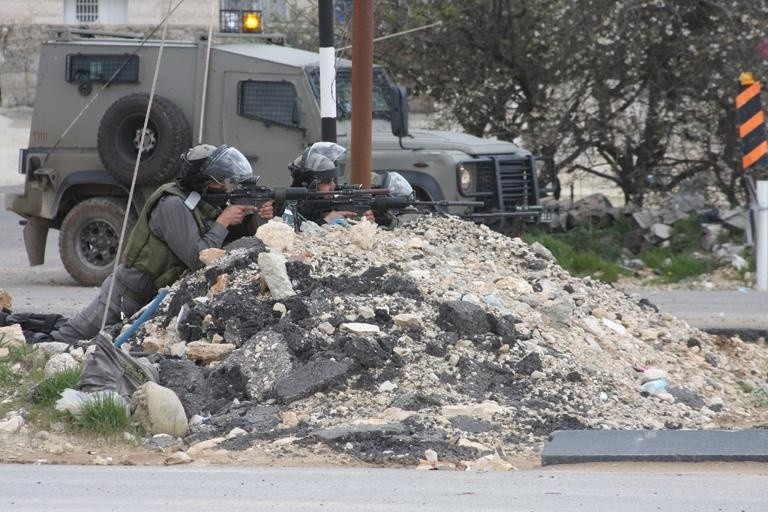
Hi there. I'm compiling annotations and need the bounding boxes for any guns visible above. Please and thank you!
[454,212,540,226]
[202,178,389,235]
[274,199,485,233]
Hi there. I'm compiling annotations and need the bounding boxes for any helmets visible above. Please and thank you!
[174,142,254,194]
[287,141,347,188]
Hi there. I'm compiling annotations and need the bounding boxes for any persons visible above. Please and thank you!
[1,144,276,346]
[282,141,378,229]
[368,169,420,223]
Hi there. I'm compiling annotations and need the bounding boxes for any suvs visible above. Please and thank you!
[4,32,558,287]
